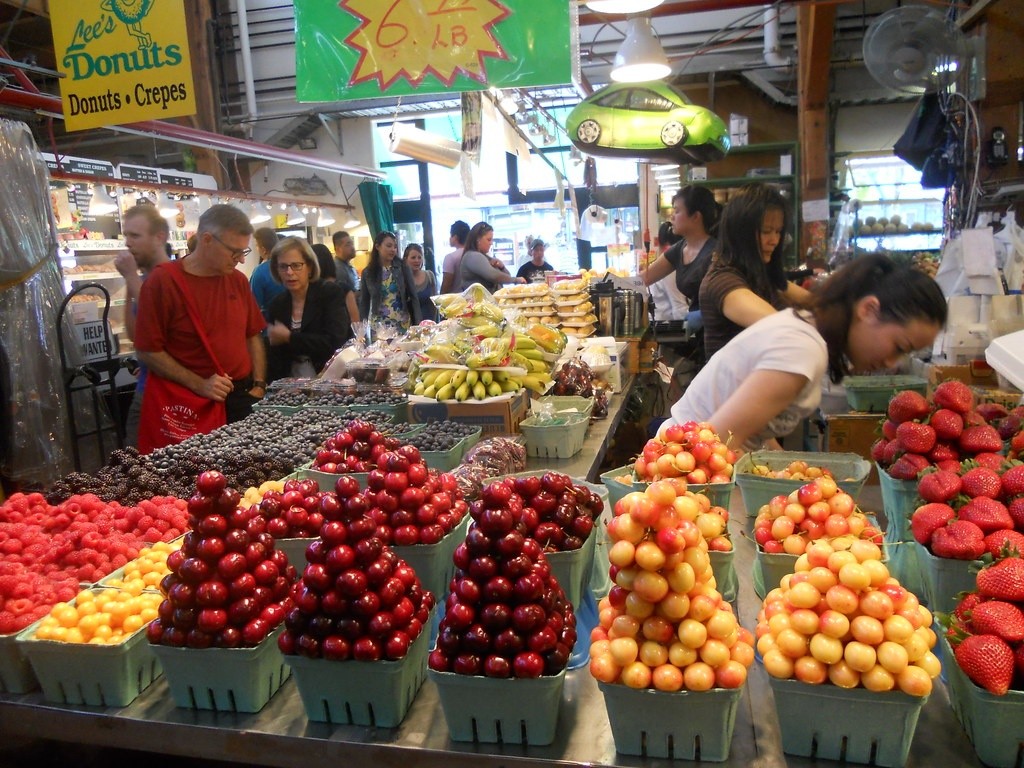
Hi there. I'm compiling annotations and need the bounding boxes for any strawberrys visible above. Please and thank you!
[875,382,1024,695]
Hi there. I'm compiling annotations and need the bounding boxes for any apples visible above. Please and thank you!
[579,267,629,280]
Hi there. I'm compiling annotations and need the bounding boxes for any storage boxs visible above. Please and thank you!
[728,118,749,144]
[687,154,792,181]
[0,337,1024,768]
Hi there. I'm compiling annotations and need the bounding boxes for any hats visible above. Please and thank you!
[530,239,544,251]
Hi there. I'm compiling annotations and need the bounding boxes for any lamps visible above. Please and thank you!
[609,10,673,84]
[66,180,361,230]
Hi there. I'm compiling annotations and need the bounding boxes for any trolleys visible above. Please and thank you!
[56,282,139,473]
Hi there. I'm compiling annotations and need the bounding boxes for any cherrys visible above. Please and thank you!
[590,420,941,696]
[148,421,604,676]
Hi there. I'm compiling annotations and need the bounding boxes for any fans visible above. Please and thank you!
[861,5,985,102]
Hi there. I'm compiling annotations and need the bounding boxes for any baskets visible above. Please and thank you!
[1,390,1024,768]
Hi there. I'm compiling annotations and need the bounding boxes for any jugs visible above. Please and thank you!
[617,287,644,338]
[590,280,617,338]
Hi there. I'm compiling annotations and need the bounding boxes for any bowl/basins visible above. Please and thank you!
[347,363,390,384]
[399,341,422,351]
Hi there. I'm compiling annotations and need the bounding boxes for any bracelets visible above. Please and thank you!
[253,381,267,391]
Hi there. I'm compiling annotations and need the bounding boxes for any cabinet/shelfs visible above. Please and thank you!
[61,238,190,335]
[684,140,802,270]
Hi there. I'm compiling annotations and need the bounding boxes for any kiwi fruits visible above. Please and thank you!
[911,251,940,279]
[850,215,934,236]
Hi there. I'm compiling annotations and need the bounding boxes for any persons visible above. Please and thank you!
[403,244,440,326]
[639,253,948,452]
[250,227,360,385]
[637,185,722,373]
[187,233,197,253]
[440,220,526,295]
[357,231,422,345]
[698,180,812,364]
[515,235,553,284]
[133,205,268,455]
[111,204,172,449]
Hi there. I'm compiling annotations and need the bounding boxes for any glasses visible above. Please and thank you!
[209,234,252,260]
[273,260,310,274]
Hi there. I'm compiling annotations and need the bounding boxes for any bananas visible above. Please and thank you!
[403,285,552,402]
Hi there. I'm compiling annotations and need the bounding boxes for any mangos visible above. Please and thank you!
[496,278,598,334]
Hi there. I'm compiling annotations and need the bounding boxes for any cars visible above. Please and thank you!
[566,79,731,166]
